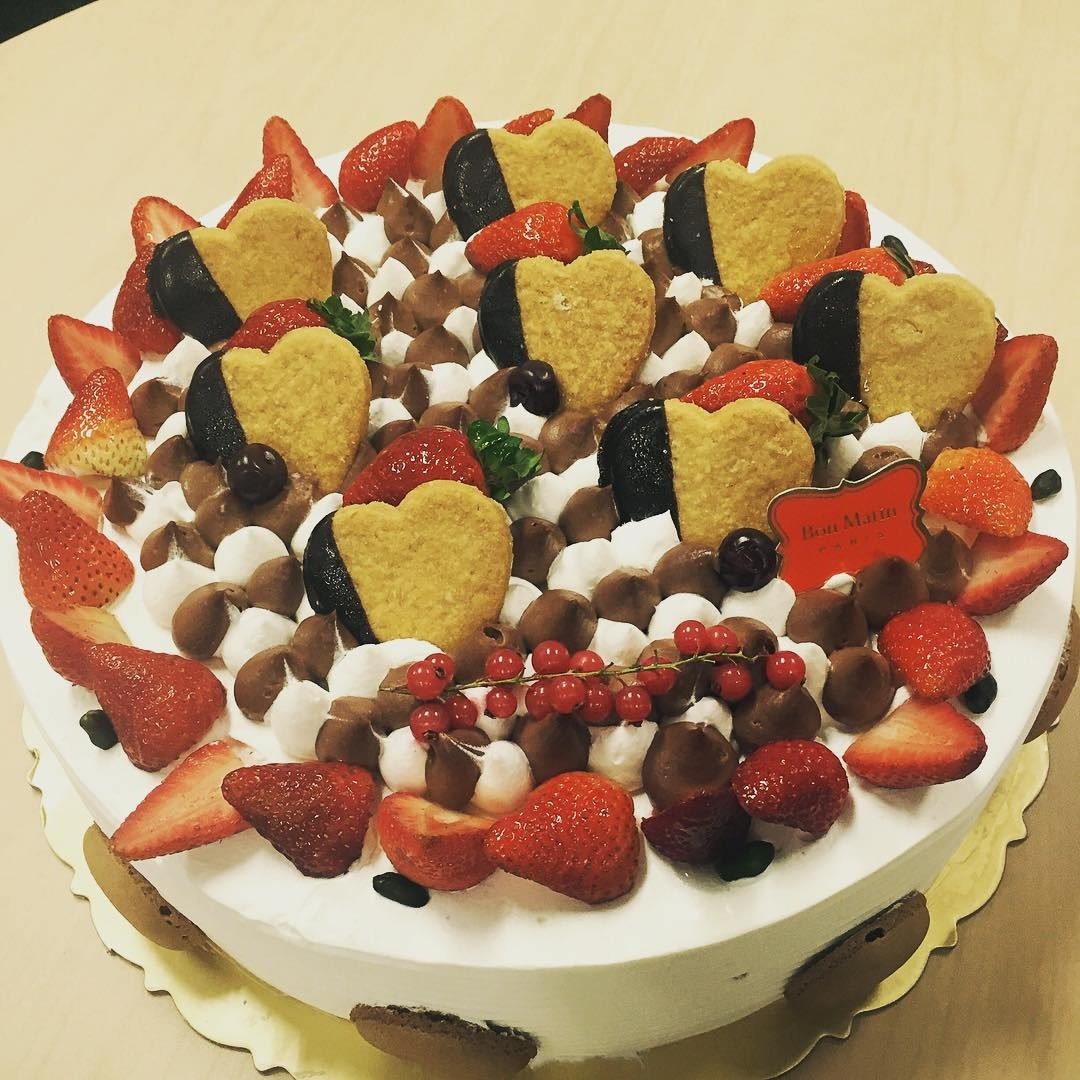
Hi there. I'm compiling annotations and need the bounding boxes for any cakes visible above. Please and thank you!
[0,94,1080,1080]
